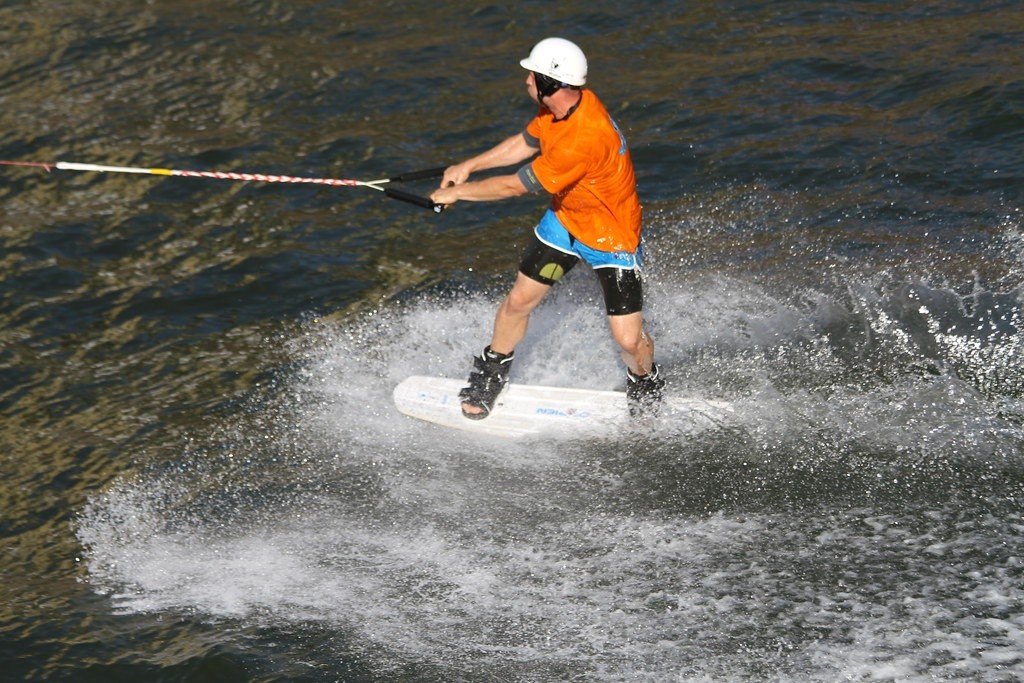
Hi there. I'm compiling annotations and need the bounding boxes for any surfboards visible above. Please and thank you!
[393,376,739,443]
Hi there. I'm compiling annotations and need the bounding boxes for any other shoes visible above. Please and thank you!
[459,345,515,420]
[626,362,666,426]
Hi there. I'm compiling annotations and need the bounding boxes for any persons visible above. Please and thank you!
[431,38,666,421]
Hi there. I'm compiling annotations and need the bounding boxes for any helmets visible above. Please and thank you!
[520,38,588,86]
